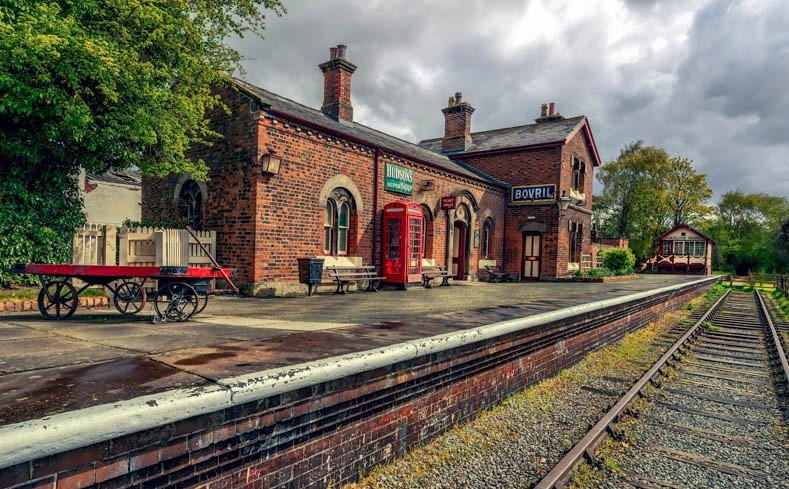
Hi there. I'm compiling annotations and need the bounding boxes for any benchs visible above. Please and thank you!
[421,264,458,288]
[484,265,521,282]
[327,265,387,294]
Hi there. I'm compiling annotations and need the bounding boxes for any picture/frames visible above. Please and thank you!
[474,230,480,248]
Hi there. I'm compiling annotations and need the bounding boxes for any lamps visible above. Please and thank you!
[556,191,572,222]
[260,149,283,175]
[528,216,535,222]
